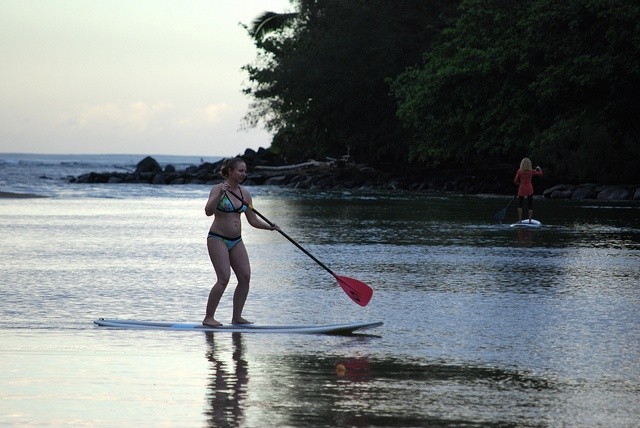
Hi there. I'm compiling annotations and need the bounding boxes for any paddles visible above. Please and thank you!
[493,167,539,224]
[227,188,373,307]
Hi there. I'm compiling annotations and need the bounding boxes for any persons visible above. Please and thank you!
[514,158,543,224]
[203,157,280,326]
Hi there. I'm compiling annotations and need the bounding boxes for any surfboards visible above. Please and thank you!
[511,218,542,228]
[94,317,386,332]
